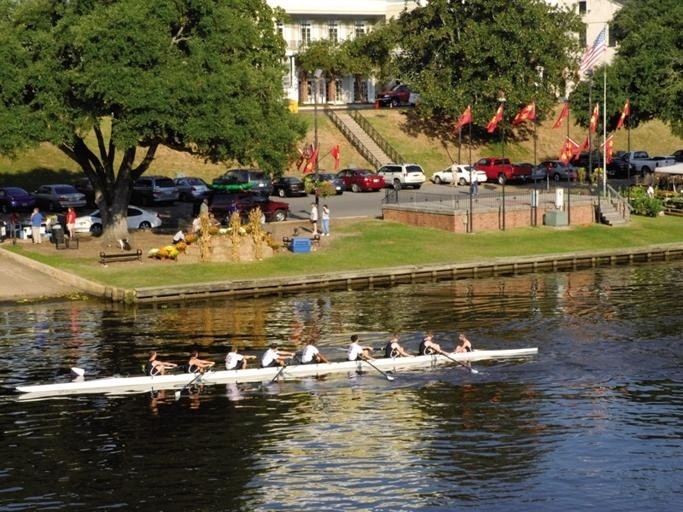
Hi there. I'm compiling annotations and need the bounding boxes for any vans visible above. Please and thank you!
[408,88,419,105]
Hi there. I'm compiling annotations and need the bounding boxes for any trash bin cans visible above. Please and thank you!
[51,223,64,243]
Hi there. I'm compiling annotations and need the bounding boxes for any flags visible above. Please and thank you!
[551,100,632,166]
[512,101,537,125]
[485,100,504,133]
[578,26,607,73]
[288,139,319,174]
[331,145,340,170]
[452,102,474,130]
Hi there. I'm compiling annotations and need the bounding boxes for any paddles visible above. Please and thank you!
[357,354,395,382]
[174,366,211,399]
[441,352,479,375]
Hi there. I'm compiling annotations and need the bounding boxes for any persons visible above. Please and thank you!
[149,390,166,414]
[469,166,480,198]
[419,333,440,355]
[449,161,459,188]
[301,339,328,365]
[185,350,215,373]
[320,205,330,237]
[261,378,289,395]
[647,181,656,201]
[223,382,247,404]
[453,333,471,352]
[226,199,239,223]
[310,202,319,236]
[385,333,410,358]
[224,347,256,370]
[147,350,177,375]
[261,343,295,368]
[185,386,206,414]
[1,202,77,245]
[199,197,210,220]
[172,229,188,245]
[347,335,374,361]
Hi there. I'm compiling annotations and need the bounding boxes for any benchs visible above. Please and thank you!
[100,248,142,264]
[282,234,320,248]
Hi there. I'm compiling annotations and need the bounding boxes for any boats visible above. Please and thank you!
[15,346,539,393]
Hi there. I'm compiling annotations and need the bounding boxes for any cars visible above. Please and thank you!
[336,168,384,193]
[0,184,37,212]
[429,163,487,186]
[30,183,90,214]
[570,150,636,181]
[536,160,577,182]
[71,202,163,235]
[513,162,546,183]
[170,176,210,201]
[272,176,306,196]
[301,172,346,195]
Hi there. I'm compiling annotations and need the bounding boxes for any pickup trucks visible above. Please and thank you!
[611,151,676,179]
[472,156,531,186]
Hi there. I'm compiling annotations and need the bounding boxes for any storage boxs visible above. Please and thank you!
[290,238,311,252]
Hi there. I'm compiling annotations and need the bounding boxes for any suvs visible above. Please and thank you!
[208,189,292,222]
[127,174,180,207]
[210,166,273,197]
[373,84,411,107]
[375,163,426,192]
